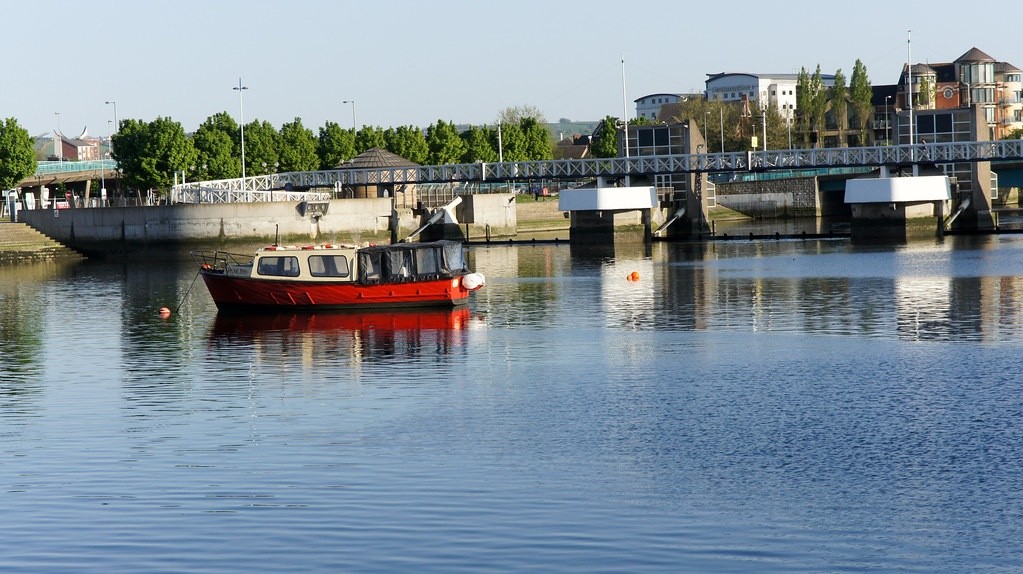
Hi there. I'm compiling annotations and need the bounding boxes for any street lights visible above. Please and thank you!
[342,100,357,136]
[107,119,112,161]
[54,112,63,168]
[105,101,117,134]
[232,76,249,179]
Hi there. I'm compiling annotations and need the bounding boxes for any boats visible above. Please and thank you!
[188,238,485,312]
[204,308,469,350]
